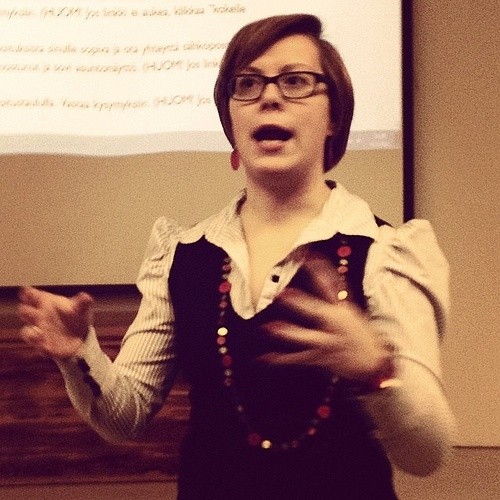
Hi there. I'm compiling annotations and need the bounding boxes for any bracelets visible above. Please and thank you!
[358,343,403,396]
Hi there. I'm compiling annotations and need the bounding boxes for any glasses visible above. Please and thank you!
[227,71,331,100]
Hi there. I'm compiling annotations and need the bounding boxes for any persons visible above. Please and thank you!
[20,14,454,500]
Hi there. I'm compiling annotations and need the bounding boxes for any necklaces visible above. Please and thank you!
[216,234,350,452]
